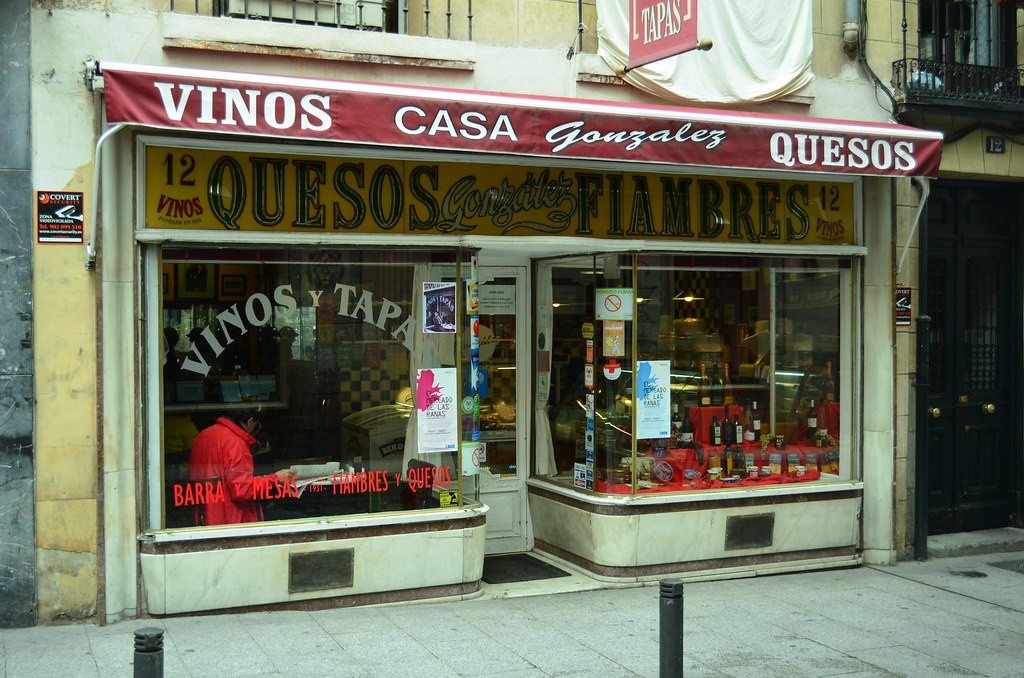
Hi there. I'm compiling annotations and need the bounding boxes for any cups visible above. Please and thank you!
[354,457,362,475]
[707,466,724,480]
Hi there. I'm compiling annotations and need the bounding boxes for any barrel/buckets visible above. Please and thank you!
[693,336,723,367]
[673,319,705,369]
[657,333,676,368]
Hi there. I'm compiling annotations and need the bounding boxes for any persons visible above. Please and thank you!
[164,412,199,479]
[187,408,297,526]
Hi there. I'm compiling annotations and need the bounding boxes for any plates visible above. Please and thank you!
[684,469,702,479]
[653,461,675,482]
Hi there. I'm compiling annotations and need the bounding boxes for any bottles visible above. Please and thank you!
[751,401,763,440]
[671,402,682,442]
[710,364,723,405]
[698,363,711,407]
[709,416,723,446]
[823,361,834,402]
[721,405,732,445]
[732,407,743,443]
[722,363,734,406]
[815,407,827,448]
[724,442,745,475]
[680,407,693,442]
[807,399,817,438]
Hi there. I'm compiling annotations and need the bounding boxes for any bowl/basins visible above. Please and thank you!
[719,477,741,483]
[653,448,666,458]
